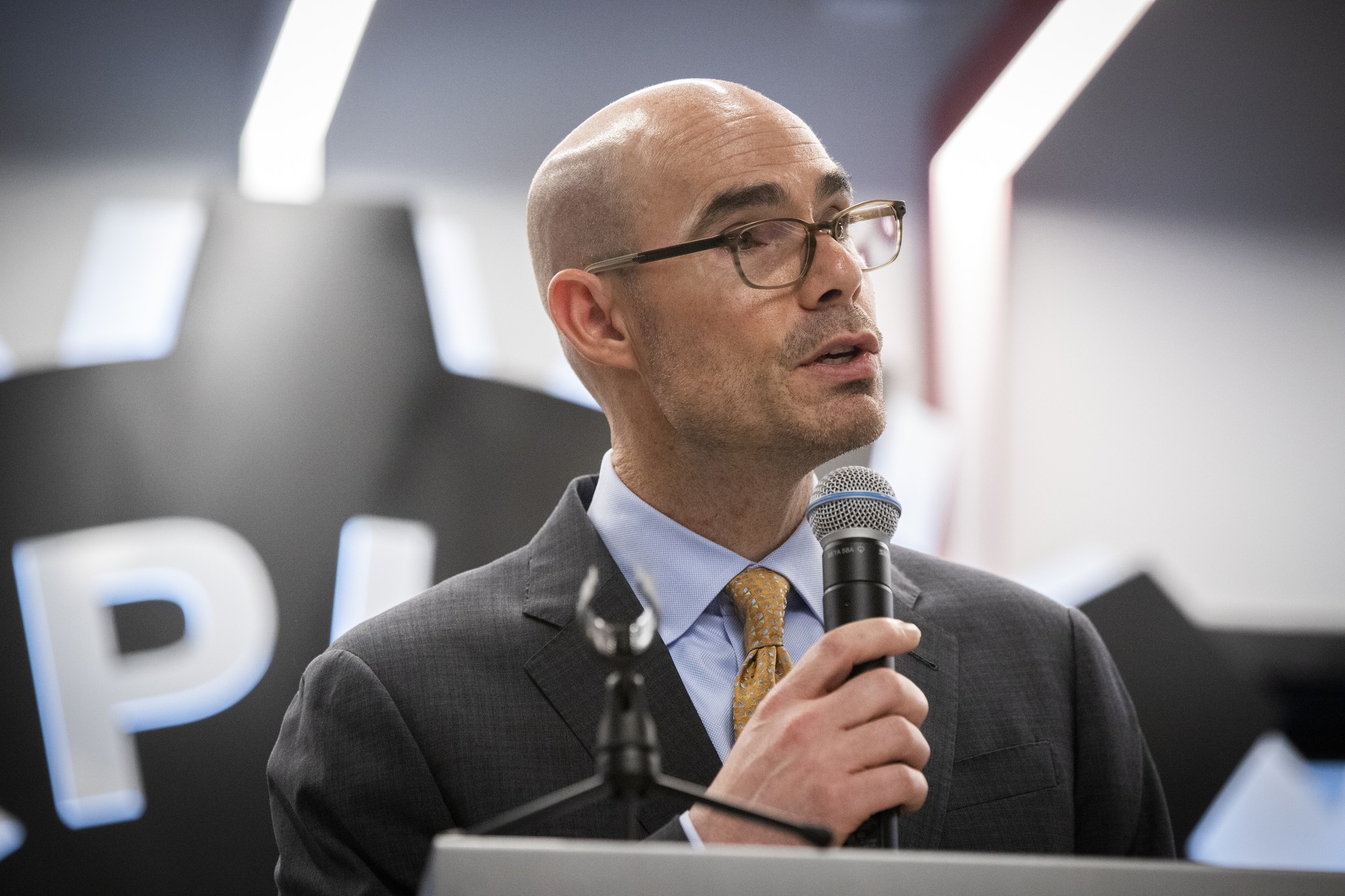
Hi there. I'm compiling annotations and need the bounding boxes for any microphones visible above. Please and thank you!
[803,464,903,850]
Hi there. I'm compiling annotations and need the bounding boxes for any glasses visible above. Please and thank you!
[586,199,906,289]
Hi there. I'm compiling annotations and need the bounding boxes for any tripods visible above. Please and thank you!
[470,566,835,847]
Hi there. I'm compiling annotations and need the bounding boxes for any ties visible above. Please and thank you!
[722,566,792,744]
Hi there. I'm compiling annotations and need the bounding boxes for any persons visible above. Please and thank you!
[267,77,1176,896]
